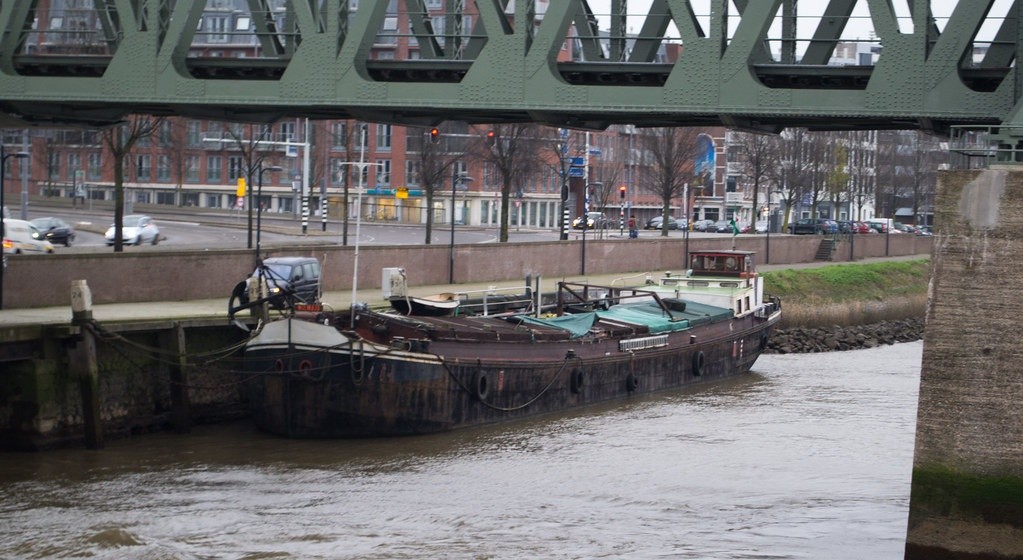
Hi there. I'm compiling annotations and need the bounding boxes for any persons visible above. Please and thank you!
[629,215,637,238]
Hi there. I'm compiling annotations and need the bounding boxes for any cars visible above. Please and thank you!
[573,212,608,230]
[0,217,55,254]
[643,215,678,231]
[104,214,161,246]
[841,217,933,237]
[675,217,770,234]
[829,220,838,233]
[29,218,76,247]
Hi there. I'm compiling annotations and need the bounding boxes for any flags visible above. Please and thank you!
[730,202,739,237]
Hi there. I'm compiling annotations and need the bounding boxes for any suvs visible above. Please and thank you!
[781,218,833,235]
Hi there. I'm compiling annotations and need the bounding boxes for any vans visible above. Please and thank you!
[244,256,322,308]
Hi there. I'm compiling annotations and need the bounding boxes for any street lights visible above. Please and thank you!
[450,160,474,285]
[1,150,32,310]
[684,179,706,269]
[886,191,901,257]
[254,160,284,270]
[765,185,782,265]
[850,192,866,261]
[582,178,605,275]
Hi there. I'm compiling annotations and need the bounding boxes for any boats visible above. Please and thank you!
[234,248,786,443]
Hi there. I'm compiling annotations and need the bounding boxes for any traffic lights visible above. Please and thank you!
[430,127,439,143]
[487,128,495,146]
[620,186,625,199]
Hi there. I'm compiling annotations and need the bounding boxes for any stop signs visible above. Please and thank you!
[237,196,245,208]
